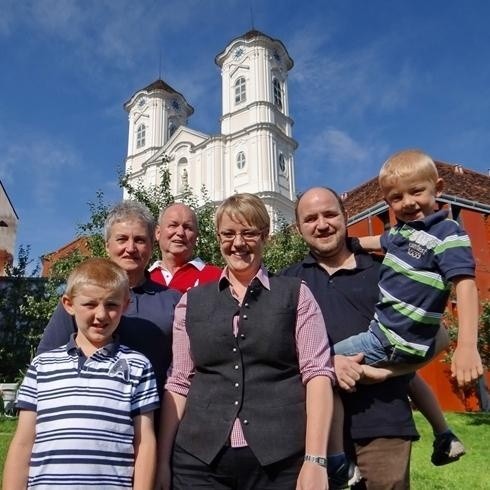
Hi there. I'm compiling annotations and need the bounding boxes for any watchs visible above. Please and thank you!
[304,454,329,467]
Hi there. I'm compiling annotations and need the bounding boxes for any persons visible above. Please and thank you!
[31,199,184,446]
[2,256,161,490]
[144,203,223,295]
[316,149,484,490]
[273,185,453,490]
[145,192,337,489]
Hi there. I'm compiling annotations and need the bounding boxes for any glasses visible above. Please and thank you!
[216,230,265,241]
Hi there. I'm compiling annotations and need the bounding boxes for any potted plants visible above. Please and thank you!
[0,347,19,418]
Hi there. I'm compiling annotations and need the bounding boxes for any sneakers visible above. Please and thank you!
[431,434,466,466]
[330,464,362,490]
